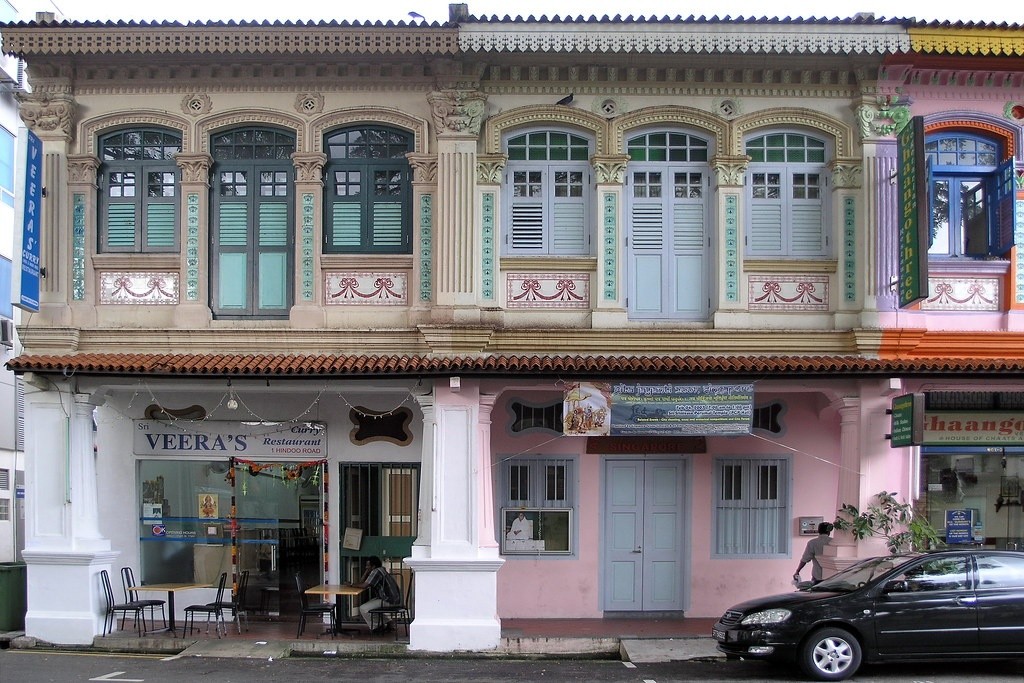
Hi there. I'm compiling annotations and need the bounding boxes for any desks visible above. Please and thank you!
[305,583,366,638]
[129,582,214,639]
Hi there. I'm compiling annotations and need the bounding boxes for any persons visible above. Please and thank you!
[506,512,530,539]
[792,521,835,586]
[346,555,401,635]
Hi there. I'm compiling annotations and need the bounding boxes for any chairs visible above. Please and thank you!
[183,572,228,639]
[294,572,414,641]
[205,570,250,635]
[100,570,154,637]
[121,567,167,631]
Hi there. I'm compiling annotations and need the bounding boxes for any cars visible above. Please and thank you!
[712,549,1024,679]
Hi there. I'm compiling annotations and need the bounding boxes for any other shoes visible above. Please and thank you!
[369,629,384,634]
[385,626,399,633]
